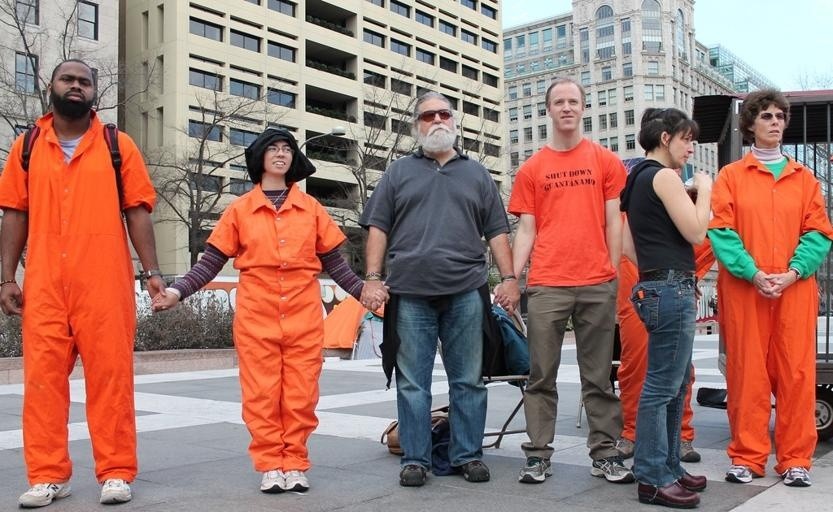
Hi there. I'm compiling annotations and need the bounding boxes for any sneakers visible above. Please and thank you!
[783,467,813,487]
[399,466,427,487]
[101,478,133,505]
[679,440,701,462]
[20,480,72,509]
[725,464,762,483]
[260,468,287,492]
[590,456,636,483]
[453,462,490,483]
[617,436,635,460]
[283,469,310,492]
[518,456,553,482]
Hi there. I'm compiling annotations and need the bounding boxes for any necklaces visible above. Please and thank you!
[272,187,287,210]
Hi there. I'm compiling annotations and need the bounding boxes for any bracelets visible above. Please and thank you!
[787,267,801,279]
[145,269,162,278]
[364,272,382,281]
[500,274,517,283]
[0,280,16,287]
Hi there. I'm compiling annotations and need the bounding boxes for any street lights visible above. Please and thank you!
[299,126,346,156]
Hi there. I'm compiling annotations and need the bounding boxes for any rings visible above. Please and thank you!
[363,300,366,304]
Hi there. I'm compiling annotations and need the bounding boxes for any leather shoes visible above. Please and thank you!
[638,481,700,508]
[677,473,707,491]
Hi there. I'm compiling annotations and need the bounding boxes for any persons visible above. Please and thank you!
[356,91,523,488]
[707,89,833,488]
[0,59,168,509]
[617,209,717,463]
[150,125,391,496]
[493,79,635,486]
[618,105,714,509]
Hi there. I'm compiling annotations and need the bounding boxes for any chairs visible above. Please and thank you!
[575,324,621,425]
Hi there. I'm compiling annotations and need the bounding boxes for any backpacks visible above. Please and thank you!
[380,416,449,455]
[490,302,530,387]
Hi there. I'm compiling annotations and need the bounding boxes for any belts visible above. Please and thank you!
[637,269,697,282]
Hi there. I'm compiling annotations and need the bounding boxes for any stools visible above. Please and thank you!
[480,372,537,451]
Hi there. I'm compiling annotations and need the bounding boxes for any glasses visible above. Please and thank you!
[264,147,295,156]
[759,114,787,120]
[414,110,453,122]
